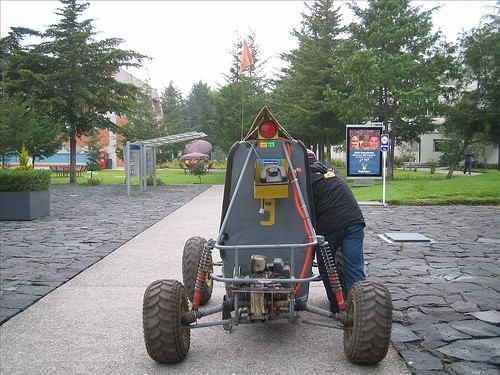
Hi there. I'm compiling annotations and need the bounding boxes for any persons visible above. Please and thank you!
[350,133,359,150]
[98,156,105,170]
[361,132,373,149]
[305,148,367,304]
[462,149,475,175]
[368,134,380,149]
[178,157,210,175]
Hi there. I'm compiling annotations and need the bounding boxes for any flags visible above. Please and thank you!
[240,41,253,72]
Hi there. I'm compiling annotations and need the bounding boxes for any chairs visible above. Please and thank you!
[180,161,213,174]
[50,165,86,177]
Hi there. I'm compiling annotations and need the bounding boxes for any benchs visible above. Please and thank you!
[401,161,434,171]
